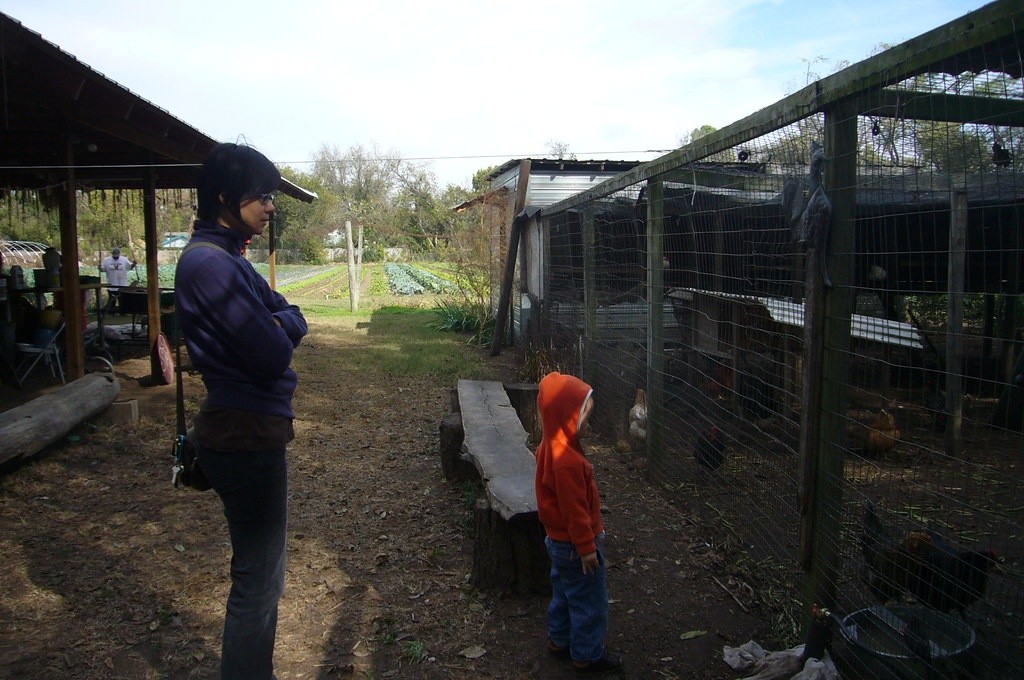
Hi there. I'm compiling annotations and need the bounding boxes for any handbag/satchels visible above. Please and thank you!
[170,425,212,492]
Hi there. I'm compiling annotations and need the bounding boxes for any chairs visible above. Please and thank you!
[14,319,66,386]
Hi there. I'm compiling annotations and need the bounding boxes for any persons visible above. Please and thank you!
[99,248,137,317]
[174,143,308,680]
[536,373,625,676]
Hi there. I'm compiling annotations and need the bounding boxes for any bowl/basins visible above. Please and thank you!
[842,601,976,679]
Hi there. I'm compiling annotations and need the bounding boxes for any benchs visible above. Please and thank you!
[439,380,541,600]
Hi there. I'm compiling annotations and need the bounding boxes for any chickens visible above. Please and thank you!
[618,362,902,476]
[862,504,1004,627]
[925,378,948,427]
[992,142,1015,170]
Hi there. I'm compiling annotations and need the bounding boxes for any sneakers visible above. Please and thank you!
[545,637,571,660]
[574,648,624,676]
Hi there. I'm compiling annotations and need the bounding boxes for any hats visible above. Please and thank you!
[113,249,120,257]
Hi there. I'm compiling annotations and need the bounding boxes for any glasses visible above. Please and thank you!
[253,193,276,205]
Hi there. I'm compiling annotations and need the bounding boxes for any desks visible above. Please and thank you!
[0,283,114,368]
